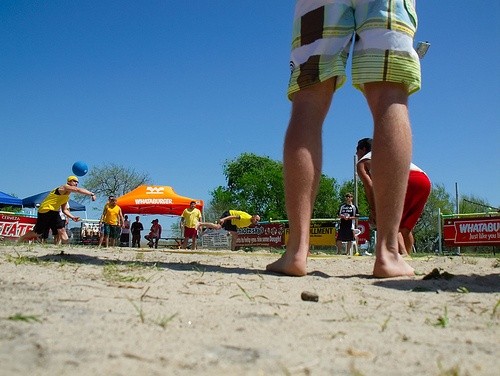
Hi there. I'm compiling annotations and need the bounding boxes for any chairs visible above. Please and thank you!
[341,228,361,256]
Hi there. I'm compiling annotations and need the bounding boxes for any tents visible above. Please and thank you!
[0,192,23,215]
[106,184,206,248]
[21,191,87,223]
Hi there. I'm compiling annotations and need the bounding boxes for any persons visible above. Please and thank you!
[151,219,161,245]
[179,201,202,250]
[40,200,105,246]
[16,176,97,244]
[264,0,427,280]
[336,193,359,255]
[144,220,159,248]
[356,138,431,260]
[119,215,130,247]
[98,196,115,248]
[195,209,260,251]
[131,216,144,248]
[101,197,126,250]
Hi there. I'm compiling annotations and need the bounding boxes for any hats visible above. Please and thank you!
[67,176,79,182]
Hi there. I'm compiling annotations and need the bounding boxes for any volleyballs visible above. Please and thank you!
[73,161,88,176]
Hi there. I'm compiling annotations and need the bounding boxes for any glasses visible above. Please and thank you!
[74,180,79,182]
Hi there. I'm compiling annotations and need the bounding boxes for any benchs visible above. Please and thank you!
[81,219,107,245]
[155,237,185,249]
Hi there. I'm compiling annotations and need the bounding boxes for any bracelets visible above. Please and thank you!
[91,193,95,196]
